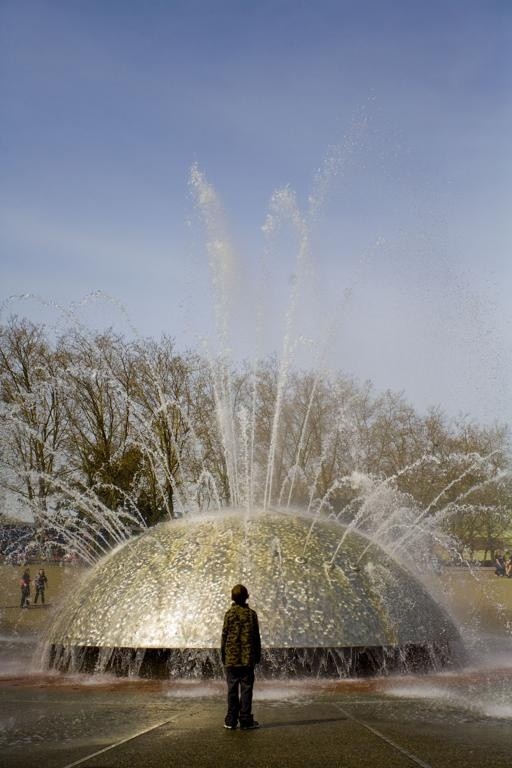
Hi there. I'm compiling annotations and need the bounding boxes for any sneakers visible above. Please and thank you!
[240,719,261,729]
[223,719,238,729]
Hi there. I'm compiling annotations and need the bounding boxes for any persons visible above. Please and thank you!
[19,567,34,608]
[220,584,260,729]
[493,549,504,576]
[33,568,48,603]
[502,547,511,577]
